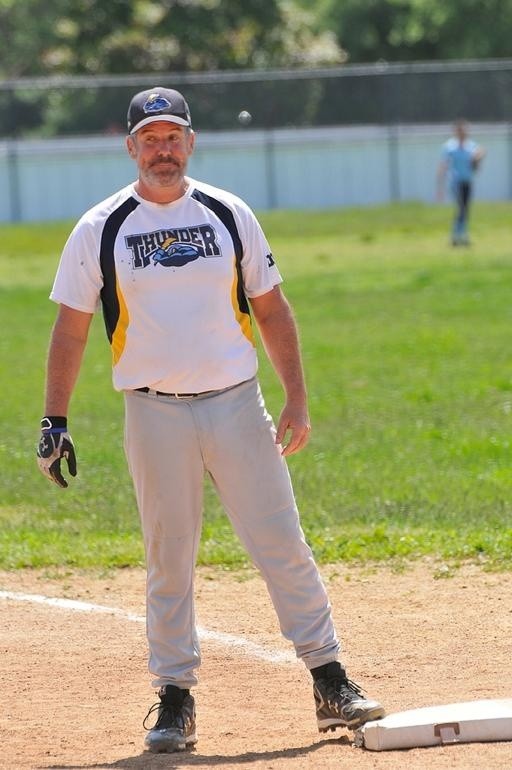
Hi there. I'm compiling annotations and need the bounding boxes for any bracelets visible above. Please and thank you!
[39,416,68,431]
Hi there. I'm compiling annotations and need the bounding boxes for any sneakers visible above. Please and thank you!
[309,661,386,733]
[144,684,199,754]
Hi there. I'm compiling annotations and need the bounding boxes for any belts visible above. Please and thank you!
[134,384,214,399]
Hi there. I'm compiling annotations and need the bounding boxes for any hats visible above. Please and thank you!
[126,86,192,135]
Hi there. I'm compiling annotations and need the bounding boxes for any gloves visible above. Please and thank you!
[36,416,77,488]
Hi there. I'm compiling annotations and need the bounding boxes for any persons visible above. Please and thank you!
[434,120,484,247]
[35,85,385,753]
